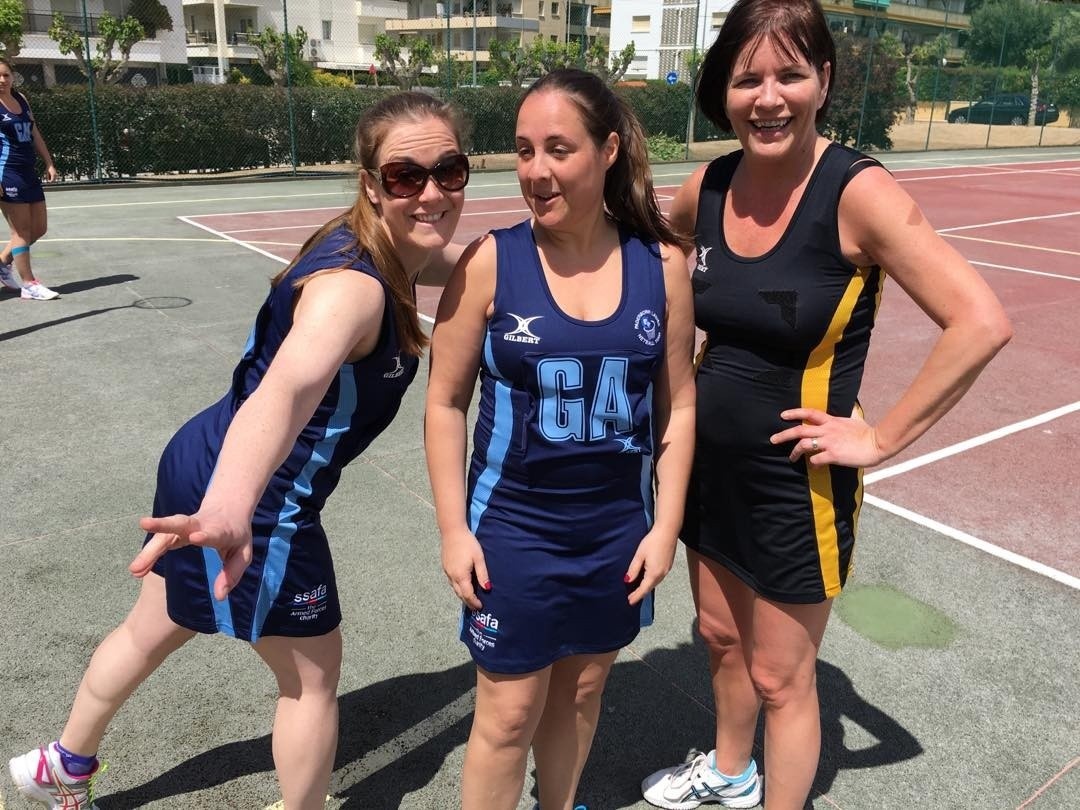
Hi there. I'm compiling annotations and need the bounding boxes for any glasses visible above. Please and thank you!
[363,153,471,199]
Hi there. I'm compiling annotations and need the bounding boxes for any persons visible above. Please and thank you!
[0,59,61,300]
[640,0,1013,810]
[9,89,471,810]
[424,70,697,810]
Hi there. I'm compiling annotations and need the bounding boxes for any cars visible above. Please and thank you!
[948,93,1059,126]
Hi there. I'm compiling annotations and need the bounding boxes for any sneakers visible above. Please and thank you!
[20,279,60,300]
[0,256,20,289]
[641,750,763,810]
[8,741,106,810]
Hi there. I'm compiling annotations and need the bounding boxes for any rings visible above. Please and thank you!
[812,438,818,452]
